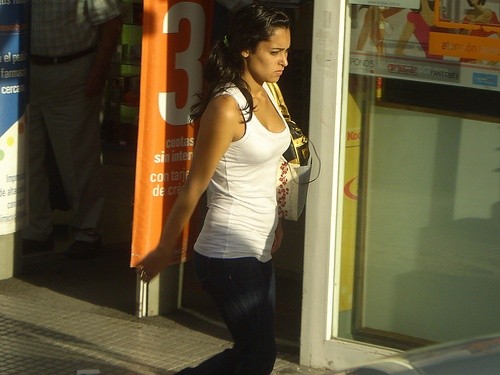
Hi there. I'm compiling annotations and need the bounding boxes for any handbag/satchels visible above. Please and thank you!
[269,82,312,222]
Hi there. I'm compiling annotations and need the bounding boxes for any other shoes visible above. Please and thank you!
[21,239,53,255]
[66,240,104,259]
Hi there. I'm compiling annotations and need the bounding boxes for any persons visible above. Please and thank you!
[134,3,290,375]
[20,0,123,258]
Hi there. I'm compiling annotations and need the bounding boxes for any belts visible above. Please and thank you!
[32,50,95,65]
